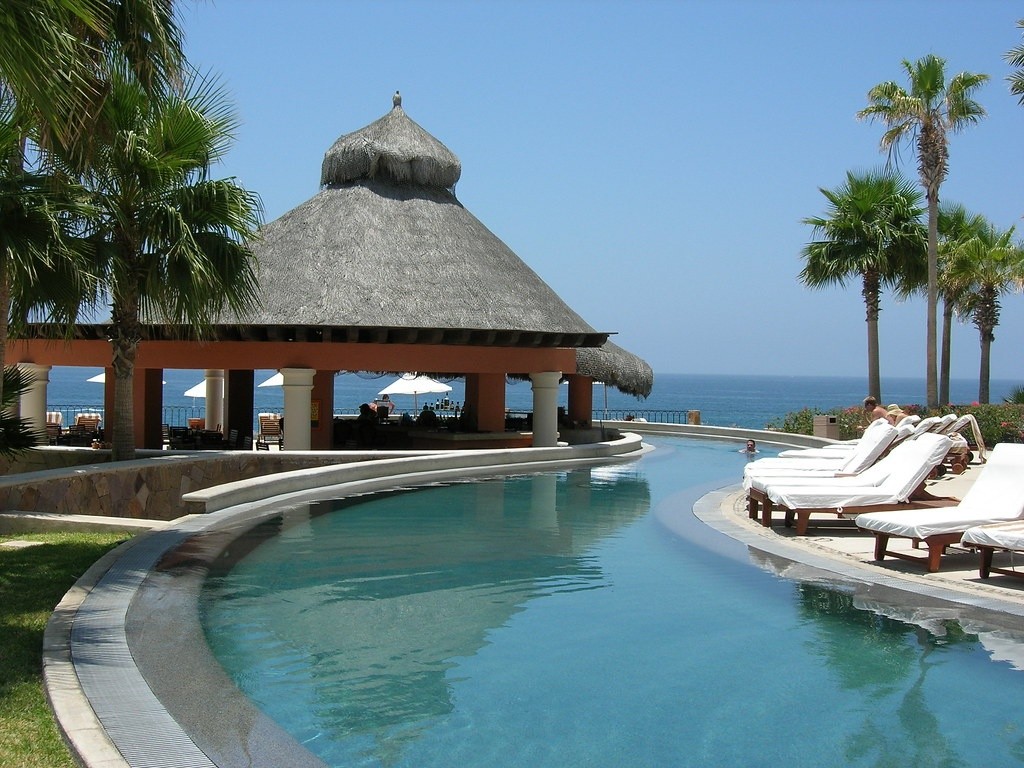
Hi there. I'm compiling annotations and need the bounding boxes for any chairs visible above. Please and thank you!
[946,413,989,465]
[741,423,899,512]
[169,427,223,450]
[932,413,957,433]
[749,439,917,529]
[959,519,1024,580]
[187,417,205,430]
[855,443,1024,573]
[743,417,889,472]
[777,424,914,459]
[221,429,239,448]
[822,414,921,450]
[162,423,171,444]
[46,411,103,447]
[255,412,284,451]
[333,401,442,447]
[766,432,953,536]
[227,435,253,450]
[915,416,943,433]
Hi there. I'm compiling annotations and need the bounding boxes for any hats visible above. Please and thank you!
[886,404,904,417]
[359,404,371,410]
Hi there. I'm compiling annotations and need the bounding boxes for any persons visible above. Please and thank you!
[419,405,436,426]
[359,395,395,421]
[458,401,477,433]
[746,440,755,452]
[862,396,908,427]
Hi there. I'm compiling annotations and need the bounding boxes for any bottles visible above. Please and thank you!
[423,403,428,410]
[436,399,440,409]
[444,392,450,410]
[430,403,434,410]
[450,401,454,410]
[456,402,459,409]
[441,399,444,409]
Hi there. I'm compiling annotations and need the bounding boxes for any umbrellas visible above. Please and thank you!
[376,371,453,416]
[86,373,166,385]
[258,371,284,387]
[184,378,225,398]
[564,379,607,409]
